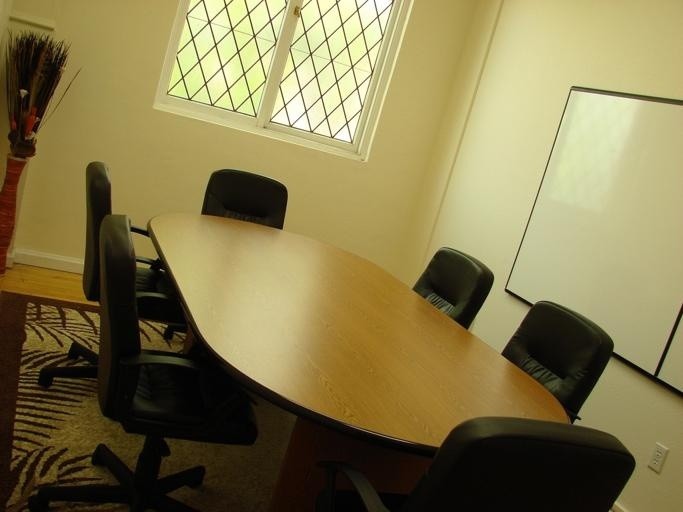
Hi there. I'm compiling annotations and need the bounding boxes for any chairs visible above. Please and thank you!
[28,161,186,388]
[412,247,496,330]
[500,299,615,420]
[201,168,290,230]
[408,415,636,511]
[28,214,261,511]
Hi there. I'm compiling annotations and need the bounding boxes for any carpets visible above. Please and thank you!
[0,287,298,512]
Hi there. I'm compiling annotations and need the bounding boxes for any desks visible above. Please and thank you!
[146,199,573,508]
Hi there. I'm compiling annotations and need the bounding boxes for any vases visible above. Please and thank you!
[0,153,28,277]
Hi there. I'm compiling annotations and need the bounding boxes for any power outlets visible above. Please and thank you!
[647,442,668,473]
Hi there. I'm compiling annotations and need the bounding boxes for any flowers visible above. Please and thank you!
[8,89,41,159]
